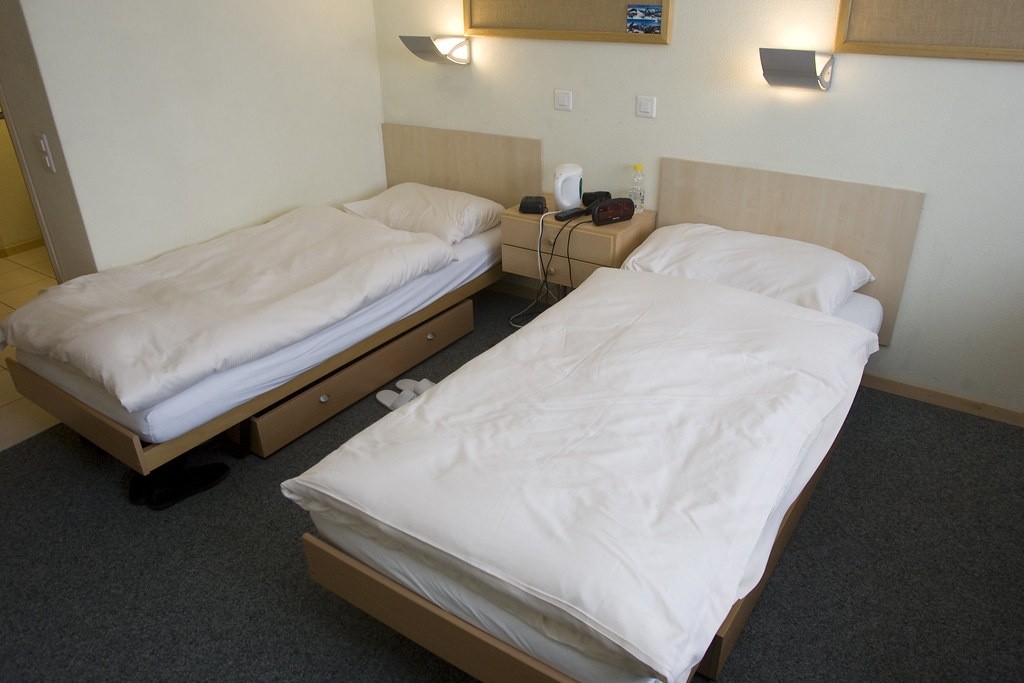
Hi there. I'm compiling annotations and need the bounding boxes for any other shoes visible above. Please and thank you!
[147,461,231,512]
[128,456,190,506]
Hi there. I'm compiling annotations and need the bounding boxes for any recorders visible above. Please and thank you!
[592,198,634,226]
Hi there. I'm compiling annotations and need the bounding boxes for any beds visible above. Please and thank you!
[302,156,926,683]
[4,123,544,477]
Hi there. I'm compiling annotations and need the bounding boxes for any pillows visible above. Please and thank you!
[620,221,875,315]
[339,182,506,247]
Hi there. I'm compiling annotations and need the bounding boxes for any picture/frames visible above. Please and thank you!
[833,0,1024,63]
[462,0,673,45]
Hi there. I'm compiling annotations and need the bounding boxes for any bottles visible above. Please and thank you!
[628,163,645,213]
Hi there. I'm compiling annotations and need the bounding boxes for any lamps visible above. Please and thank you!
[758,46,835,92]
[398,32,473,67]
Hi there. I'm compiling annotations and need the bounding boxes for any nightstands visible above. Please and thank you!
[500,193,657,291]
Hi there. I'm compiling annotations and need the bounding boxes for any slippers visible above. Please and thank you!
[395,378,437,395]
[376,390,419,412]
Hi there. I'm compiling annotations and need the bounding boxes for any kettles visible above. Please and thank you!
[553,163,583,211]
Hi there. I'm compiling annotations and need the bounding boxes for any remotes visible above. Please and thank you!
[555,208,585,221]
